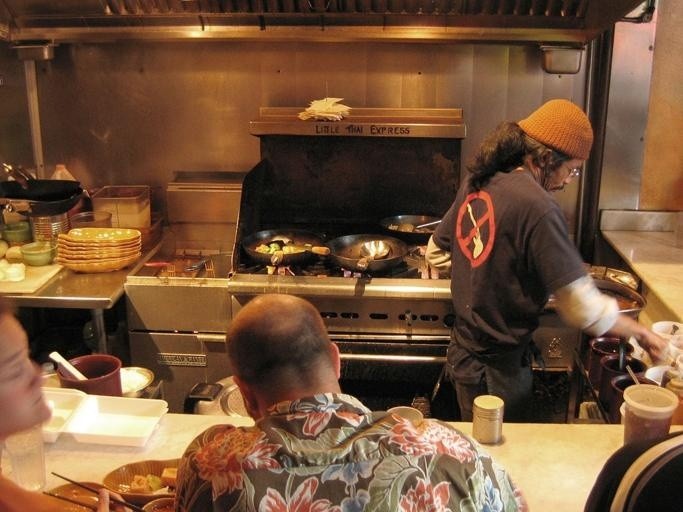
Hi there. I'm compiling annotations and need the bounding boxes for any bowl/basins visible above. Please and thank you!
[120,365,154,397]
[32,217,70,241]
[2,221,32,242]
[20,241,57,267]
[69,212,112,226]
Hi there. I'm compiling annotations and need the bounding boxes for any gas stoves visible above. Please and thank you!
[235,221,454,280]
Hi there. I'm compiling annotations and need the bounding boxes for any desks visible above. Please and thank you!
[0,222,176,358]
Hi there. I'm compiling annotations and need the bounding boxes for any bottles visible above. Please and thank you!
[472,395,505,444]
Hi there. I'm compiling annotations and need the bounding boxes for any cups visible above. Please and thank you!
[6,421,46,492]
[622,384,679,441]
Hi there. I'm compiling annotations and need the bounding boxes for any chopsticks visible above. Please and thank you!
[43,471,148,512]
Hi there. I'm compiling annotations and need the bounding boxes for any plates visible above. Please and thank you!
[39,482,127,512]
[103,459,178,504]
[57,227,142,273]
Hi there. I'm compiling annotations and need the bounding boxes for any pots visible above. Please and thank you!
[378,213,443,244]
[241,227,329,268]
[0,162,84,216]
[545,279,647,321]
[326,234,407,275]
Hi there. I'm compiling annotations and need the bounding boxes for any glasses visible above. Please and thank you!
[562,162,580,177]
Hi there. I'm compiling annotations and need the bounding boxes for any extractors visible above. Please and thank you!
[0,0,657,75]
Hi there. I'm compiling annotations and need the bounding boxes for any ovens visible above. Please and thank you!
[330,333,578,423]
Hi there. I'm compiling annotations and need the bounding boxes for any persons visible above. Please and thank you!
[584,433,683,512]
[173,294,530,511]
[274,135,457,237]
[0,294,134,511]
[423,98,670,422]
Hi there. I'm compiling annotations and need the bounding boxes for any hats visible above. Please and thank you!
[517,98,594,160]
